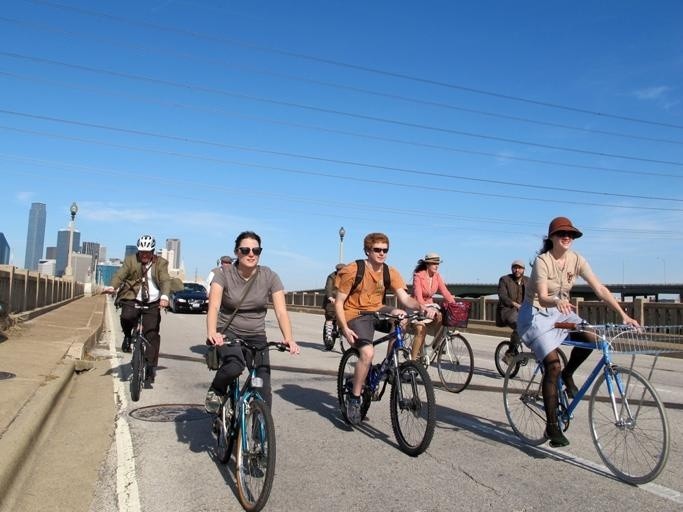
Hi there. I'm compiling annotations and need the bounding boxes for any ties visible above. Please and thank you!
[141,264,148,301]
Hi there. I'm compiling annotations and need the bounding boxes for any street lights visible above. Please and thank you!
[216,258,220,266]
[62,201,79,277]
[655,255,666,284]
[336,226,346,264]
[92,249,103,286]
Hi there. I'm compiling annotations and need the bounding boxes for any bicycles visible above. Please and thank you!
[499,318,683,488]
[322,305,363,357]
[204,339,297,512]
[394,299,474,393]
[113,295,170,401]
[493,316,571,379]
[334,308,446,456]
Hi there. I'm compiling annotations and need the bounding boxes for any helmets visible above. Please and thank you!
[136,235,155,252]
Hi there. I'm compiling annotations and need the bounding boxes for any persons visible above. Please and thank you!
[517,217,642,446]
[100,235,171,382]
[207,256,232,294]
[322,263,347,338]
[403,252,454,361]
[335,233,439,425]
[495,260,530,365]
[207,230,301,467]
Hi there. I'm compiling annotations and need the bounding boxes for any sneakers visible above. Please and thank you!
[204,391,222,414]
[346,391,361,424]
[388,372,418,384]
[254,444,267,468]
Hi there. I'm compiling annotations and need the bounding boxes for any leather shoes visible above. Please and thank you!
[566,380,578,399]
[122,337,131,352]
[543,423,568,447]
[145,369,153,383]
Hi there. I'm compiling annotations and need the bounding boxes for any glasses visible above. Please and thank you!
[555,231,572,238]
[235,247,262,255]
[370,248,387,253]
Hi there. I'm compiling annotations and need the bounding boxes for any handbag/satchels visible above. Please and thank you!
[443,302,470,326]
[205,346,219,370]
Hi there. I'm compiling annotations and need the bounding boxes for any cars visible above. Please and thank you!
[165,278,210,313]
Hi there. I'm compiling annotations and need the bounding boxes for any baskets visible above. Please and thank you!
[441,305,471,328]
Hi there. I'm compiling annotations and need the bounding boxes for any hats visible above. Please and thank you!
[221,256,232,262]
[417,254,442,264]
[511,260,524,269]
[335,264,345,269]
[548,216,582,238]
